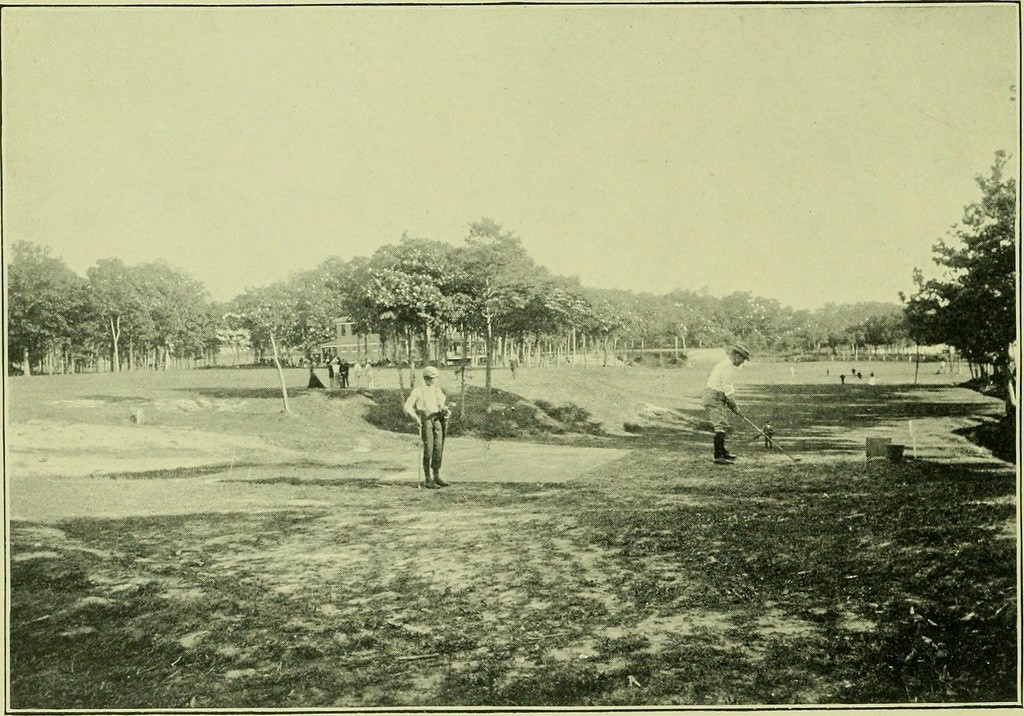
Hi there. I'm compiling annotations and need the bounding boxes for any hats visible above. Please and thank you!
[732,342,752,362]
[423,366,440,378]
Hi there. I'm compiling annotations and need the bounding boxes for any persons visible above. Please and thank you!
[702,341,750,464]
[403,366,451,488]
[328,359,373,389]
[763,421,774,449]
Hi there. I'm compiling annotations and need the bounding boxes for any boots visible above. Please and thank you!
[713,434,738,465]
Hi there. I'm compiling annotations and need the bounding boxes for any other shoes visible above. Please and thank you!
[426,474,449,489]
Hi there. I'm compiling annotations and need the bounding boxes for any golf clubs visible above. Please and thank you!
[417,414,422,491]
[732,407,802,462]
